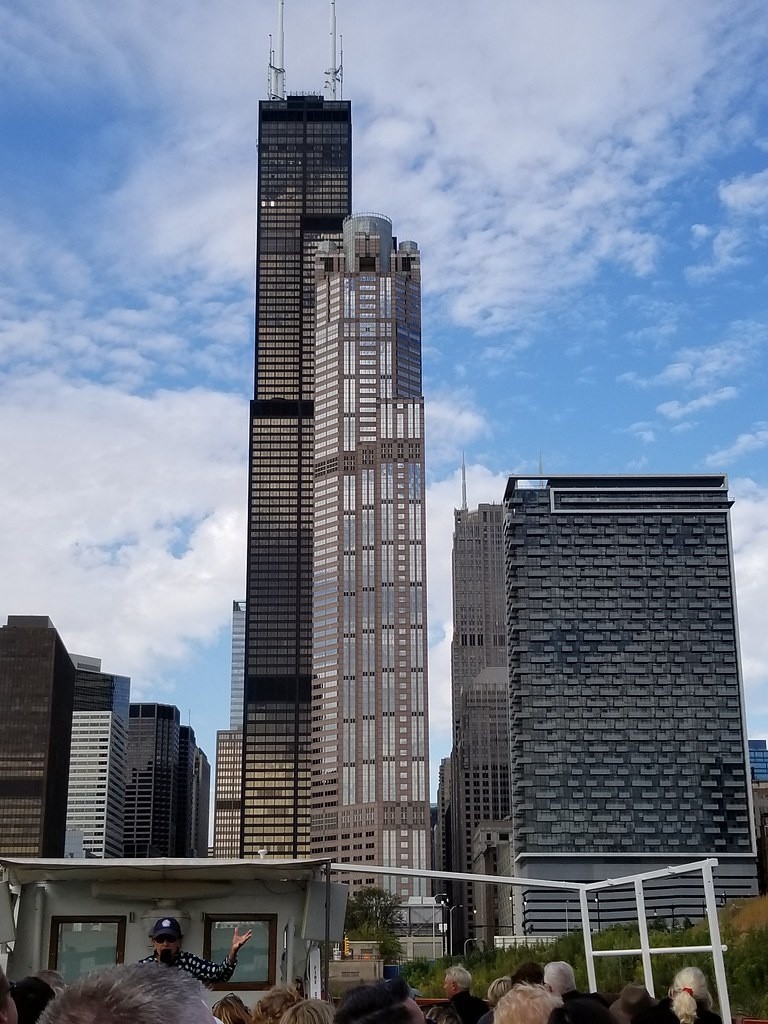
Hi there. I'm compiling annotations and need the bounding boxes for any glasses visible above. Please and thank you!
[6,980,17,995]
[154,934,177,943]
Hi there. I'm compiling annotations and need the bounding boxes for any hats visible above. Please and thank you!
[152,916,181,940]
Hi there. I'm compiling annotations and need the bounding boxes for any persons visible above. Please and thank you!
[0,962,661,1024]
[443,967,490,1024]
[654,967,722,1024]
[136,917,252,984]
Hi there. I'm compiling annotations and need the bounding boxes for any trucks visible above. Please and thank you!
[0,854,336,1017]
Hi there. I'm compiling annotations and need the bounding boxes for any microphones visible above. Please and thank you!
[160,949,172,966]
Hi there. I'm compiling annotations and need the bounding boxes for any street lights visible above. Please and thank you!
[652,904,676,925]
[522,896,527,936]
[509,887,514,937]
[720,891,727,904]
[464,937,478,956]
[595,892,601,932]
[433,893,447,961]
[442,903,464,956]
